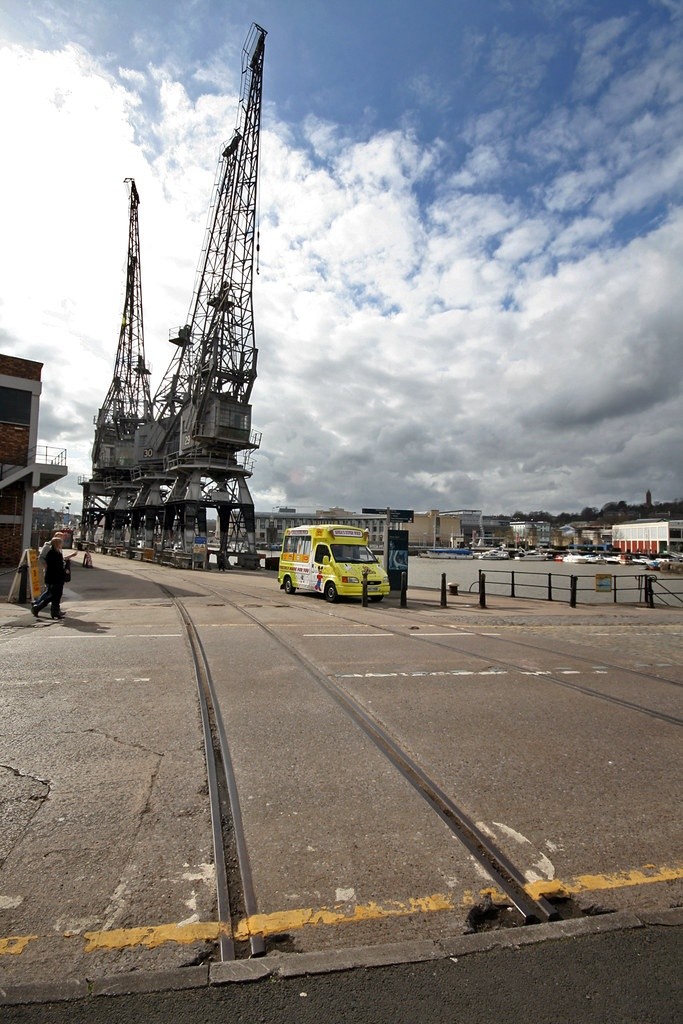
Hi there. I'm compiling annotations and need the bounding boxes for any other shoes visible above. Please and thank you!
[52,614,64,619]
[59,611,66,616]
[31,606,38,617]
[30,601,36,606]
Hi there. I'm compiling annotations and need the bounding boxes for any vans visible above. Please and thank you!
[279,524,393,606]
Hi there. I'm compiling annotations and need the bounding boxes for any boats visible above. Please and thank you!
[555,546,683,570]
[513,546,553,562]
[473,548,510,561]
[418,552,429,559]
[427,549,473,560]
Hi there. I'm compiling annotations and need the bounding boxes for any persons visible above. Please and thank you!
[30,537,66,620]
[29,532,68,614]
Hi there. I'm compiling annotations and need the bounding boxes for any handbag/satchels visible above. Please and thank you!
[63,560,72,583]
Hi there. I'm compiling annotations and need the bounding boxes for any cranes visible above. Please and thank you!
[70,22,270,576]
